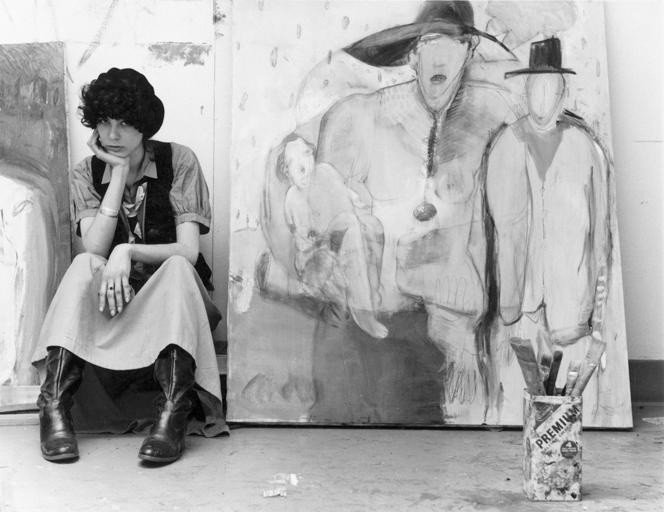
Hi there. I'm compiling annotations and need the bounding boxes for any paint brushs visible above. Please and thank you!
[538,336,608,398]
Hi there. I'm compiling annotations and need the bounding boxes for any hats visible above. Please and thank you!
[343,0,517,66]
[503,37,576,78]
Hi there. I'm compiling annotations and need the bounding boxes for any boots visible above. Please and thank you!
[138,344,196,463]
[38,346,84,462]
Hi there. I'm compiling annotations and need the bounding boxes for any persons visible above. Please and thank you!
[31,66,232,465]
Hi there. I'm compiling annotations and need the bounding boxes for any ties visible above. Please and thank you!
[121,182,143,275]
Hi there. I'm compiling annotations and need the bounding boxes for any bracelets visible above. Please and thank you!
[98,206,120,218]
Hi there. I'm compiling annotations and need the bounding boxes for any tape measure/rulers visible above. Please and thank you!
[509,338,548,395]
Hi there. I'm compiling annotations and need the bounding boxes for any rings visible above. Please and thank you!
[107,286,115,291]
